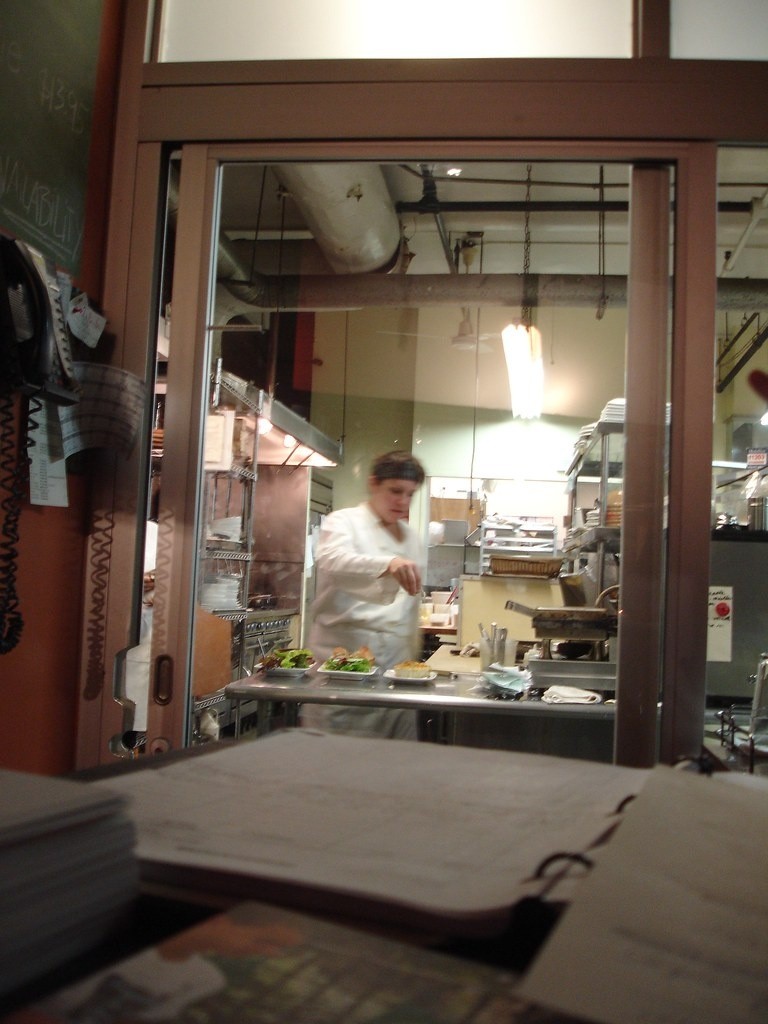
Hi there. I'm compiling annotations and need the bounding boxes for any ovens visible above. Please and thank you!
[239,630,293,705]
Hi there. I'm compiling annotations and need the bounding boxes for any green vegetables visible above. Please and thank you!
[326,659,368,672]
[276,649,313,667]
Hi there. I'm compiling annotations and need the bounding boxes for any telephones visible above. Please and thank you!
[0,236,81,408]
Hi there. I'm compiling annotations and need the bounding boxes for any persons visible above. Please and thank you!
[299,450,428,741]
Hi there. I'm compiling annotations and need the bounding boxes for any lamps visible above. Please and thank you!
[500,162,544,420]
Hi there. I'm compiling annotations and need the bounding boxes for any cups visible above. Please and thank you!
[419,591,458,626]
[748,496,768,532]
[481,639,519,673]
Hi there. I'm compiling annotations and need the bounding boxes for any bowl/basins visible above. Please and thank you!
[605,490,622,527]
[207,516,242,541]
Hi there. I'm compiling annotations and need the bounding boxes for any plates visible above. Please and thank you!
[573,422,596,448]
[254,662,318,678]
[599,398,625,423]
[665,402,670,425]
[383,670,437,685]
[317,661,380,681]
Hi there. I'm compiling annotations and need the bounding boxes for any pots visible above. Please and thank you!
[248,593,278,607]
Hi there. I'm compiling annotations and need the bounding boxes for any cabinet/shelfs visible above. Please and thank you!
[185,354,265,748]
[564,422,623,660]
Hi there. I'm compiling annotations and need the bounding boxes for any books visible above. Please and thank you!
[0,895,537,1023]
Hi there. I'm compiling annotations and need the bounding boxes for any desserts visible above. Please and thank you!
[395,661,430,677]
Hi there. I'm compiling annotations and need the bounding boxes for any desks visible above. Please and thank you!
[0,738,603,1024]
[225,674,615,740]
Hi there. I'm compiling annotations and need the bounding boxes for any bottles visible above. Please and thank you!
[199,708,220,741]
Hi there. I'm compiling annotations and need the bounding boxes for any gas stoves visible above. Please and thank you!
[245,607,300,637]
